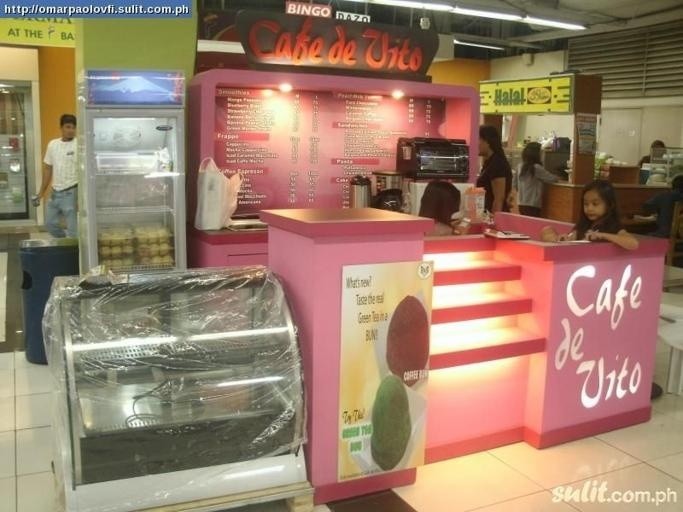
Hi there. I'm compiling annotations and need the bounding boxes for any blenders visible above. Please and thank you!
[350,175,371,209]
[462,187,487,235]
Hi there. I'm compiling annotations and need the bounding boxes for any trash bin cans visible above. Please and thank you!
[19,238,79,364]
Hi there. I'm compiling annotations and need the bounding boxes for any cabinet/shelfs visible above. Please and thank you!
[483,181,674,235]
[259,201,671,505]
[499,145,573,181]
[187,220,268,268]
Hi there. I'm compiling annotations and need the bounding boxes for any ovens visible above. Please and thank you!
[395,136,471,180]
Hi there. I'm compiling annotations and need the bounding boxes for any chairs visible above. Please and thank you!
[663,201,682,292]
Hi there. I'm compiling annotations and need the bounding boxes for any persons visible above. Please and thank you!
[643,173,683,239]
[513,142,559,216]
[561,178,639,249]
[33,114,78,238]
[477,125,512,213]
[637,140,669,168]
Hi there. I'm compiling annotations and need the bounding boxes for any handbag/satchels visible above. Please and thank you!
[193,158,244,229]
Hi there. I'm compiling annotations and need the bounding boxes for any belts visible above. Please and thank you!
[62,183,78,192]
